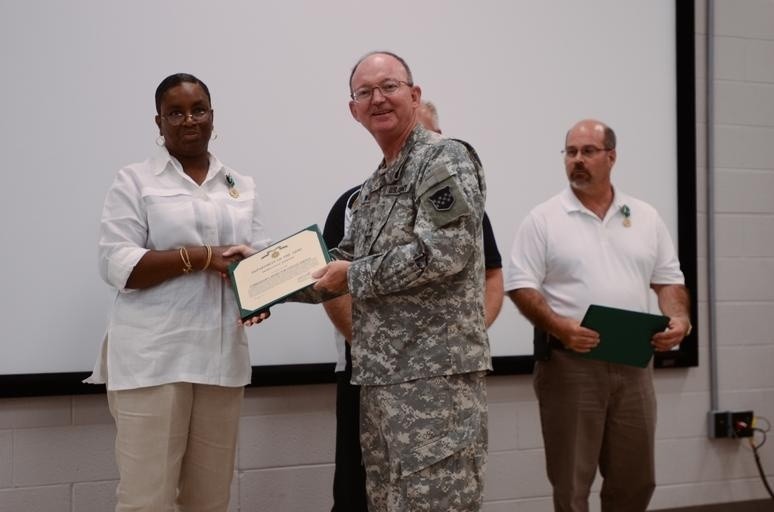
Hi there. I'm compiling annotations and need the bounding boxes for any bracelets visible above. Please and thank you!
[177,246,194,276]
[687,324,691,337]
[200,244,213,271]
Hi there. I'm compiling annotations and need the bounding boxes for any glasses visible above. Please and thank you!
[561,145,612,157]
[352,80,413,104]
[158,109,213,128]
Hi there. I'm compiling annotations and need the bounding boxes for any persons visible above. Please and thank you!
[502,118,693,512]
[219,48,496,512]
[318,100,505,512]
[96,73,273,512]
